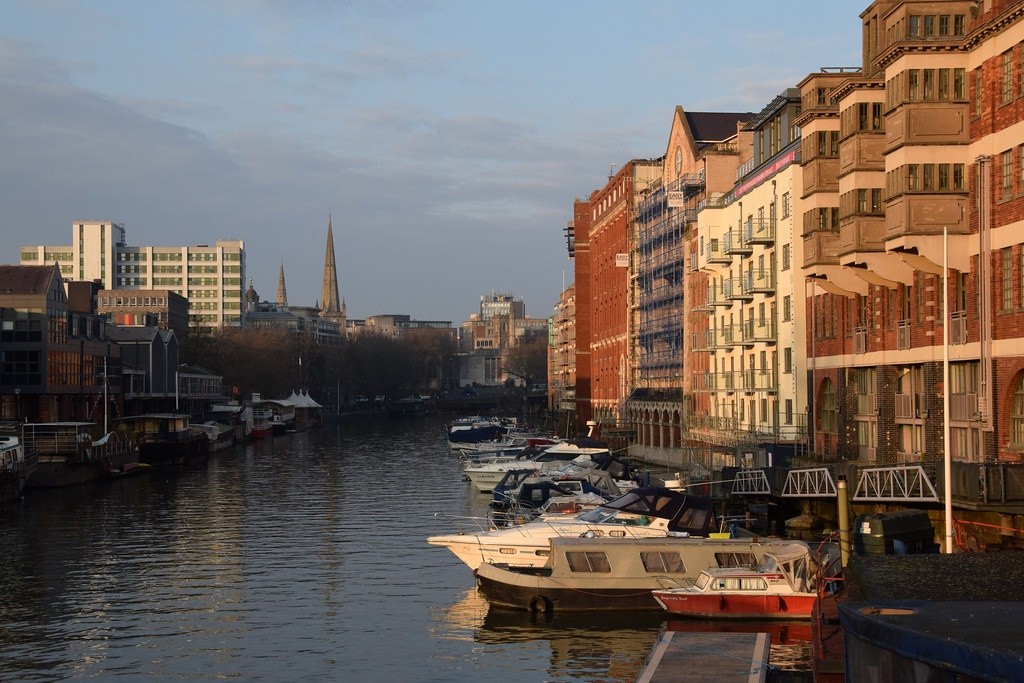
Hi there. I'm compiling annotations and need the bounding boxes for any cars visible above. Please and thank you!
[354,388,480,403]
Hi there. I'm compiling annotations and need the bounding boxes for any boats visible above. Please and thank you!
[648,542,847,621]
[477,533,821,612]
[0,355,326,504]
[424,487,764,589]
[445,414,650,522]
